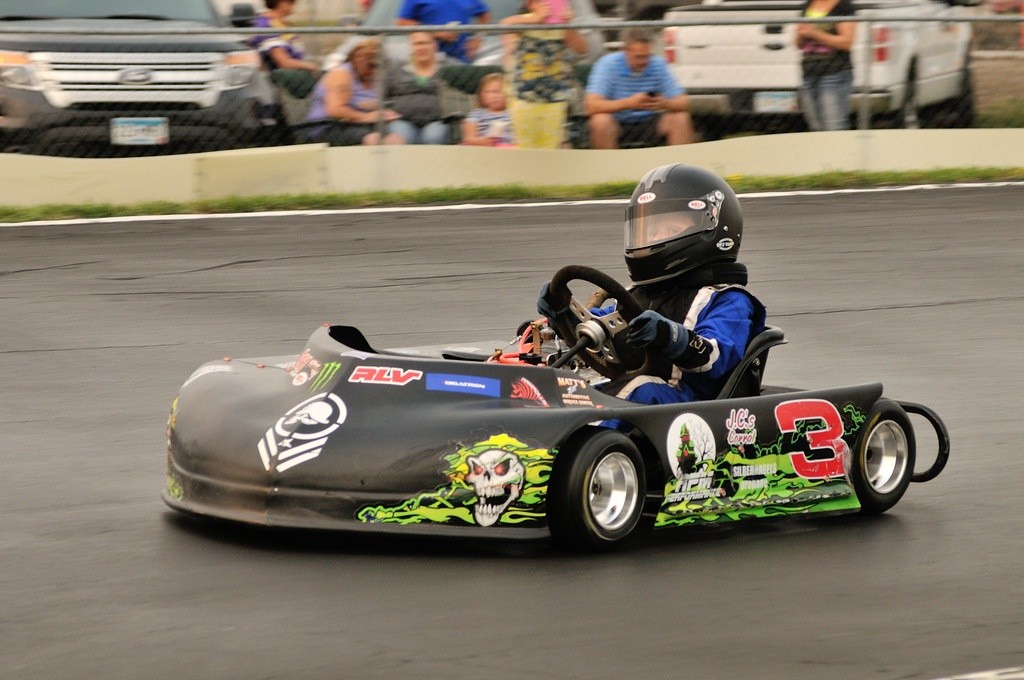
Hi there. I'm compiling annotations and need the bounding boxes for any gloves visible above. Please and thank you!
[626,308,690,357]
[536,281,573,328]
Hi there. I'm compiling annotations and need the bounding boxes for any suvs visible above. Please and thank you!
[0,1,279,157]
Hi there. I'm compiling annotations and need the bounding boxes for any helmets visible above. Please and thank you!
[622,162,743,285]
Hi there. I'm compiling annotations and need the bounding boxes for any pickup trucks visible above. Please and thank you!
[662,0,981,137]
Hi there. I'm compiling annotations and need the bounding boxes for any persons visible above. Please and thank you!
[537,164,766,428]
[392,0,489,65]
[583,27,692,149]
[460,70,513,146]
[797,0,857,132]
[306,38,403,145]
[247,0,320,129]
[382,31,508,145]
[497,0,594,149]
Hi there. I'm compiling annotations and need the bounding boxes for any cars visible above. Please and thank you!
[320,0,622,74]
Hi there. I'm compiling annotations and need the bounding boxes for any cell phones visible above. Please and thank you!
[648,91,657,96]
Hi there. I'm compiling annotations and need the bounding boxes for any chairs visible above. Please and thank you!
[702,324,792,400]
[574,63,653,148]
